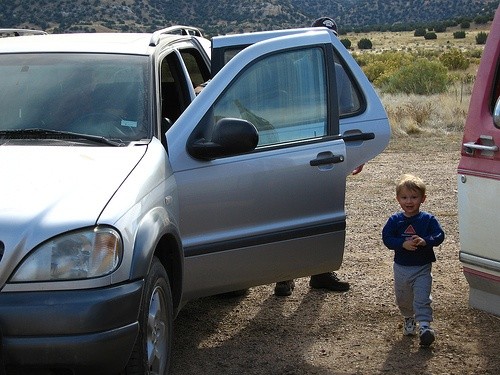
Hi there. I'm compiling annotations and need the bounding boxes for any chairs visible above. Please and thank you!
[92,81,177,118]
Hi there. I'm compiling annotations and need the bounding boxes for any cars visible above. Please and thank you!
[456,3,500,316]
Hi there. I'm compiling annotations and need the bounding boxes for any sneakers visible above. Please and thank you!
[402,316,417,337]
[418,325,436,346]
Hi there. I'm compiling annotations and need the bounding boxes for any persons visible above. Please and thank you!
[195,16,366,298]
[382,174,445,346]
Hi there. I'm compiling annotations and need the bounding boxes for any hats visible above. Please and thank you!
[311,17,338,35]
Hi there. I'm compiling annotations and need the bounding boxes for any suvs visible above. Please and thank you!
[0,26,389,372]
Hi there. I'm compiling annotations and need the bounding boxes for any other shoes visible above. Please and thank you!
[309,271,350,292]
[275,279,295,295]
